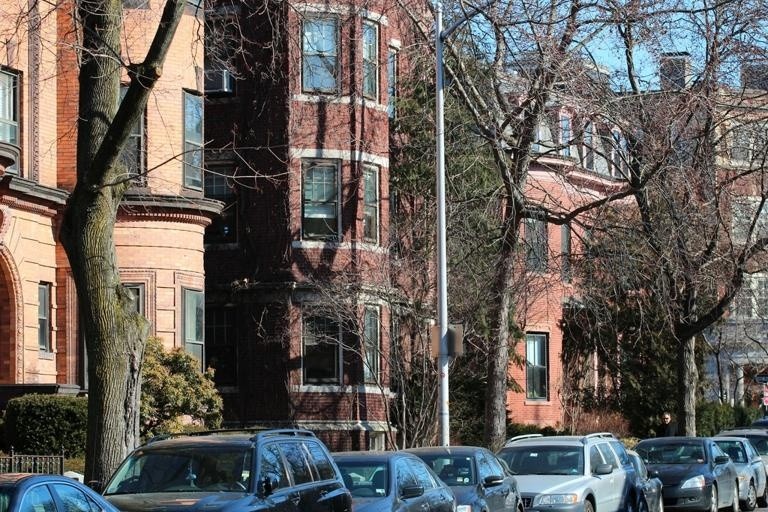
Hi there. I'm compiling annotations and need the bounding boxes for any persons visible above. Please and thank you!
[656,411,679,437]
[539,426,555,436]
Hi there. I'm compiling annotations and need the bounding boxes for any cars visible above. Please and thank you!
[1,473,119,512]
[329,450,457,511]
[400,446,526,511]
[102,427,353,511]
[626,414,768,512]
[494,433,638,511]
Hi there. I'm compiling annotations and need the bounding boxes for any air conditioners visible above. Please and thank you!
[203,68,232,94]
